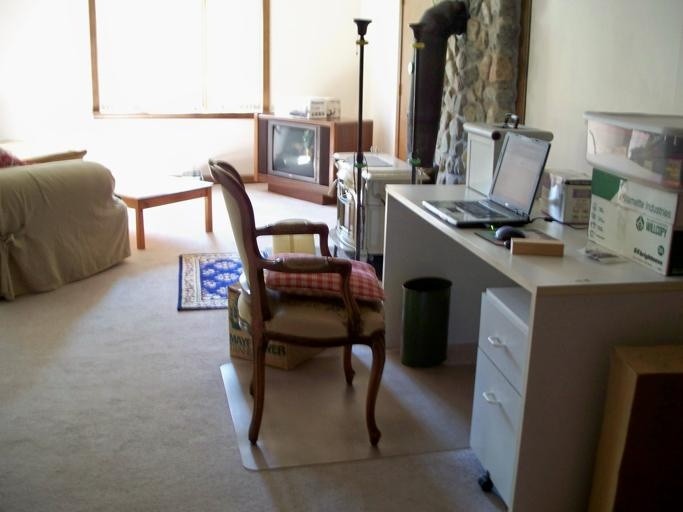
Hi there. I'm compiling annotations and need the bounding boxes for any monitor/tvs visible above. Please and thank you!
[268,119,320,184]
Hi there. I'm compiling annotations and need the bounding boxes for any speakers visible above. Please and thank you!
[258,118,268,174]
[320,127,330,186]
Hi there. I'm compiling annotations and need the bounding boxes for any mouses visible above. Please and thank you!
[495,225,525,240]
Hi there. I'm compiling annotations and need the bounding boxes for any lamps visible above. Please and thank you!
[353,19,426,260]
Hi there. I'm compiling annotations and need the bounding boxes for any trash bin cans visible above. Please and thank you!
[400,276,452,368]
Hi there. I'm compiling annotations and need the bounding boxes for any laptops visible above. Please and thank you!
[422,131,551,228]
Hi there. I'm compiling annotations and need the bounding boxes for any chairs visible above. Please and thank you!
[210,158,385,449]
[1,157,128,300]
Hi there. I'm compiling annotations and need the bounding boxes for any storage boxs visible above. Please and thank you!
[583,111,683,192]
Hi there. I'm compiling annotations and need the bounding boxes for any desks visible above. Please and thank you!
[115,174,214,249]
[384,183,682,512]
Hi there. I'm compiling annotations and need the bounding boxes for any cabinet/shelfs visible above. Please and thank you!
[334,150,412,256]
[259,107,373,205]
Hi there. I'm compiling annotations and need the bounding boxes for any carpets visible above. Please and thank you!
[219,341,477,470]
[179,253,270,312]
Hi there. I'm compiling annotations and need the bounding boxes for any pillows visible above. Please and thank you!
[0,146,86,167]
[266,247,385,304]
